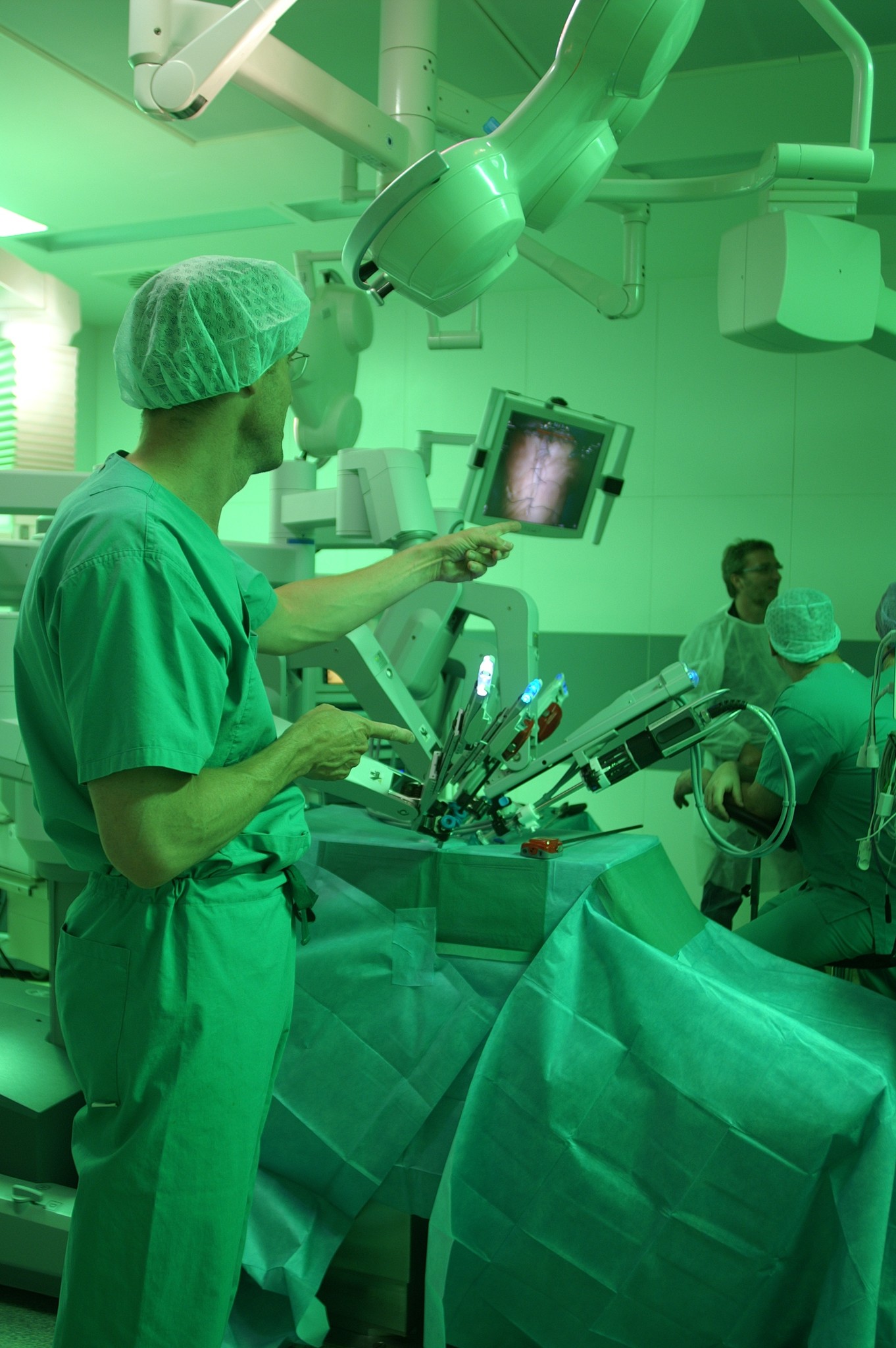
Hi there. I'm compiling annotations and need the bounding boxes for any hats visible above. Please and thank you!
[764,587,842,664]
[874,583,896,648]
[111,255,312,410]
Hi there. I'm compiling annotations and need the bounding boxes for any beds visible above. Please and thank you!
[521,760,767,925]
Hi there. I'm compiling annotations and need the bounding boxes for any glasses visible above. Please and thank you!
[287,347,312,383]
[743,561,784,575]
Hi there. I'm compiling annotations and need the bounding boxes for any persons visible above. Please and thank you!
[678,538,794,930]
[864,581,896,697]
[9,253,523,1348]
[673,587,896,969]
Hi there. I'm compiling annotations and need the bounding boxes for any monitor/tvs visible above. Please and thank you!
[461,385,635,545]
